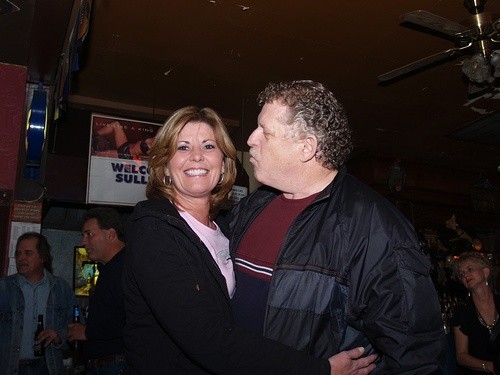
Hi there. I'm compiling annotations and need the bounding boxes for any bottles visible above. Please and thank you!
[72,305,83,359]
[34,314,45,357]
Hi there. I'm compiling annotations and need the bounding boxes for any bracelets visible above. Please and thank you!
[482,360,487,372]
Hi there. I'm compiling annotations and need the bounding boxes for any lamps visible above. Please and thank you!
[461,44,500,83]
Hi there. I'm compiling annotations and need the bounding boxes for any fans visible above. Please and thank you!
[375,0,500,83]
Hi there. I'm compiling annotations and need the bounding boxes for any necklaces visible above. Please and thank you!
[475,306,498,333]
[209,217,212,228]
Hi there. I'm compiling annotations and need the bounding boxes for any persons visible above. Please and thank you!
[452,252,500,375]
[121,107,378,375]
[223,79,448,375]
[93,120,155,160]
[67,208,126,375]
[0,232,85,375]
